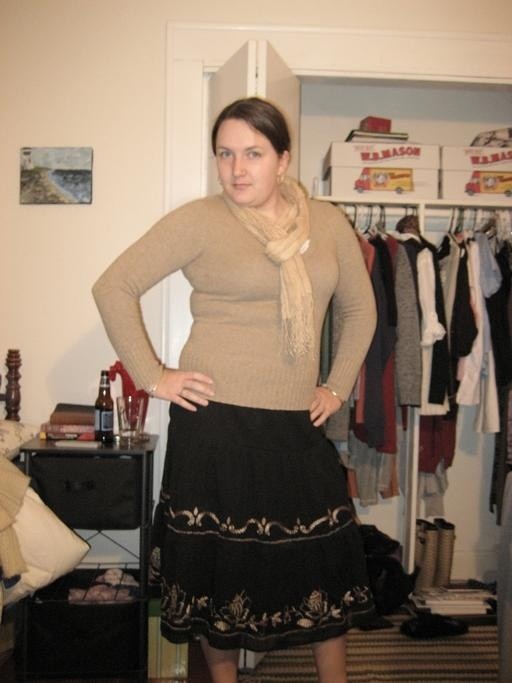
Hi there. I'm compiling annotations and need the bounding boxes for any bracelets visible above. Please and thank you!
[320,383,346,412]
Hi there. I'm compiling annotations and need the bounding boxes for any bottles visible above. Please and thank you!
[92,370,114,443]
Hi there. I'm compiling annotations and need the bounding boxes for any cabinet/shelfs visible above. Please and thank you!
[16,429,159,681]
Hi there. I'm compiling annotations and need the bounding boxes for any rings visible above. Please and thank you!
[177,386,186,396]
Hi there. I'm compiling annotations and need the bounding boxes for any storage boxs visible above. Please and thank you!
[320,140,511,204]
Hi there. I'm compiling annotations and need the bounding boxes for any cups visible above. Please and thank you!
[117,397,145,442]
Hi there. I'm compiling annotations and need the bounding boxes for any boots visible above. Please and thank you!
[433,518,456,589]
[412,518,439,596]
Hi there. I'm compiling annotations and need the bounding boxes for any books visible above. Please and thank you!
[401,583,499,617]
[37,401,97,448]
[343,129,410,144]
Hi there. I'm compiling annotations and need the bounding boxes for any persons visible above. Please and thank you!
[90,95,377,683]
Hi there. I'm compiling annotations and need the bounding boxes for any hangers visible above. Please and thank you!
[431,203,466,261]
